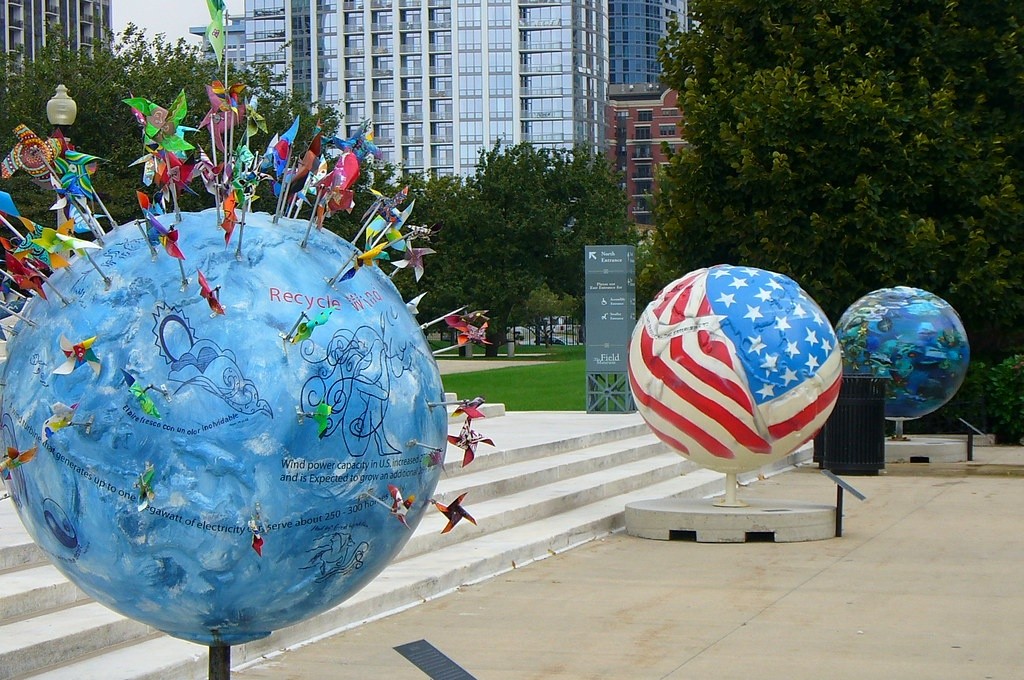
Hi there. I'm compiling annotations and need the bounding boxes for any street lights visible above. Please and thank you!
[46,83,78,255]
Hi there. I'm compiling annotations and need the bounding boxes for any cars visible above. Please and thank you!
[532,336,577,345]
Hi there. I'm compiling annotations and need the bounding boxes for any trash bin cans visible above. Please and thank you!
[823,376,891,476]
[812,373,875,469]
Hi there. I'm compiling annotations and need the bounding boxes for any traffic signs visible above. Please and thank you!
[584,246,637,273]
